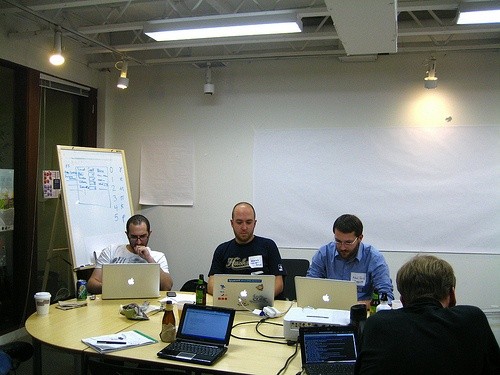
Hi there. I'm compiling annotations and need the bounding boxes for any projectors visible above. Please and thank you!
[283,307,350,343]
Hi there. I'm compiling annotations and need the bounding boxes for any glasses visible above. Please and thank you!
[127,233,149,241]
[333,237,358,247]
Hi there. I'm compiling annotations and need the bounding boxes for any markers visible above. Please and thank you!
[84,264,94,266]
[93,251,98,262]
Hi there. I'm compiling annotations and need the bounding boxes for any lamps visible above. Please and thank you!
[203,63,214,95]
[50,30,65,66]
[423,55,439,82]
[116,60,130,90]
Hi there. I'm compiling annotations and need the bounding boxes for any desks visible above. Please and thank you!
[24,291,195,375]
[84,292,302,375]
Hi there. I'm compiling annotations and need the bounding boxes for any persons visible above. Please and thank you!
[87,214,173,375]
[354,253,500,375]
[306,214,395,305]
[207,202,286,300]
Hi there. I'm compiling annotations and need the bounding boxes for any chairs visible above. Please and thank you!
[281,259,310,302]
[180,279,208,293]
[0,341,34,375]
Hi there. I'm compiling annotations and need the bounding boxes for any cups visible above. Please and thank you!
[391,300,403,310]
[177,300,194,320]
[34,292,51,316]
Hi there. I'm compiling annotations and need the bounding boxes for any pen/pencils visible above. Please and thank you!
[97,340,126,345]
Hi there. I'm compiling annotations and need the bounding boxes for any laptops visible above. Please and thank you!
[101,263,160,300]
[157,303,235,365]
[298,325,360,375]
[213,274,275,311]
[294,276,357,311]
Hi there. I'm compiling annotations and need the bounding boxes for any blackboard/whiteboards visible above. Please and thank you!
[55,144,135,270]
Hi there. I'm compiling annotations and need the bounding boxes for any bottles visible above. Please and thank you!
[162,301,176,342]
[350,304,367,340]
[369,294,380,315]
[376,292,392,313]
[196,274,206,305]
